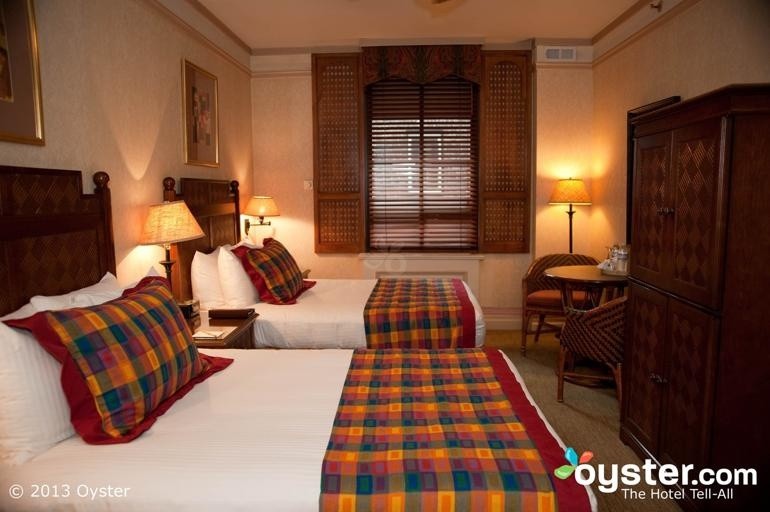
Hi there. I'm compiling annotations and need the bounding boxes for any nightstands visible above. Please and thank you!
[301,268,311,279]
[192,312,260,347]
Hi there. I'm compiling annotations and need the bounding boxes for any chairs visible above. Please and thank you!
[557,296,627,405]
[520,253,602,359]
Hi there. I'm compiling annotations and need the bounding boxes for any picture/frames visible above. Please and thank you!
[181,57,220,168]
[0,1,45,147]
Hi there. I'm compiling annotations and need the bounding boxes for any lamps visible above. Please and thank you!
[549,177,593,253]
[138,200,206,294]
[243,195,280,236]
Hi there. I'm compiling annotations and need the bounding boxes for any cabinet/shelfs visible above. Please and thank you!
[619,82,770,512]
[359,253,484,300]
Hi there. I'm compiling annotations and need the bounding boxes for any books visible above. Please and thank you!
[207,308,254,321]
[191,331,224,340]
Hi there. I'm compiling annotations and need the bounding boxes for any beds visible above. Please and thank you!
[542,265,628,389]
[0,166,598,512]
[163,175,486,348]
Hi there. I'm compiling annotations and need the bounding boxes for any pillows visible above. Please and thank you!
[223,239,247,252]
[230,238,317,305]
[70,264,160,307]
[30,270,119,312]
[2,276,235,446]
[218,248,259,308]
[191,245,224,310]
[0,321,76,469]
[0,304,33,321]
